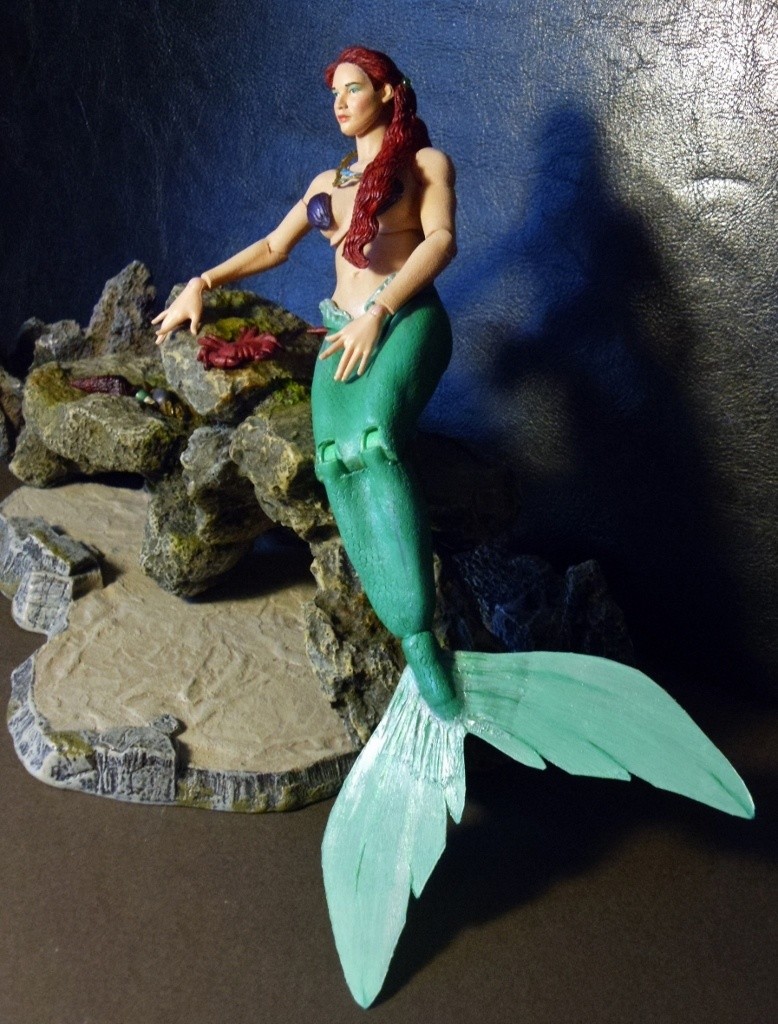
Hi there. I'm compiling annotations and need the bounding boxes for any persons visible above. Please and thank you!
[151,46,757,1008]
